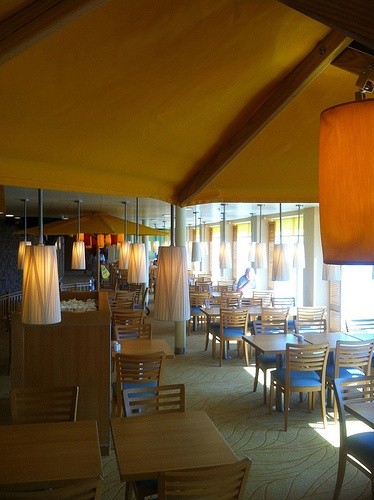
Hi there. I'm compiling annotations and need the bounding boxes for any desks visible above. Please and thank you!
[200,306,226,351]
[109,409,240,500]
[293,333,365,375]
[0,421,104,500]
[118,338,176,380]
[242,334,314,405]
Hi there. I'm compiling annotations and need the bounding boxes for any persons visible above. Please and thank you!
[88,248,106,288]
[236,267,256,293]
[153,255,158,265]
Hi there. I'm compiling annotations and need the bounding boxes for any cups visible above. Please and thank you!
[114,344,118,352]
[117,344,120,350]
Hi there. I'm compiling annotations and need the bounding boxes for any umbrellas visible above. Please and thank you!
[0,0,374,207]
[15,212,169,290]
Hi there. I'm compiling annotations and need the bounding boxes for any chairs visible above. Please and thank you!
[9,264,374,500]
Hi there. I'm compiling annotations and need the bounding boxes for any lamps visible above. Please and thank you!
[318,97,374,266]
[18,189,339,325]
